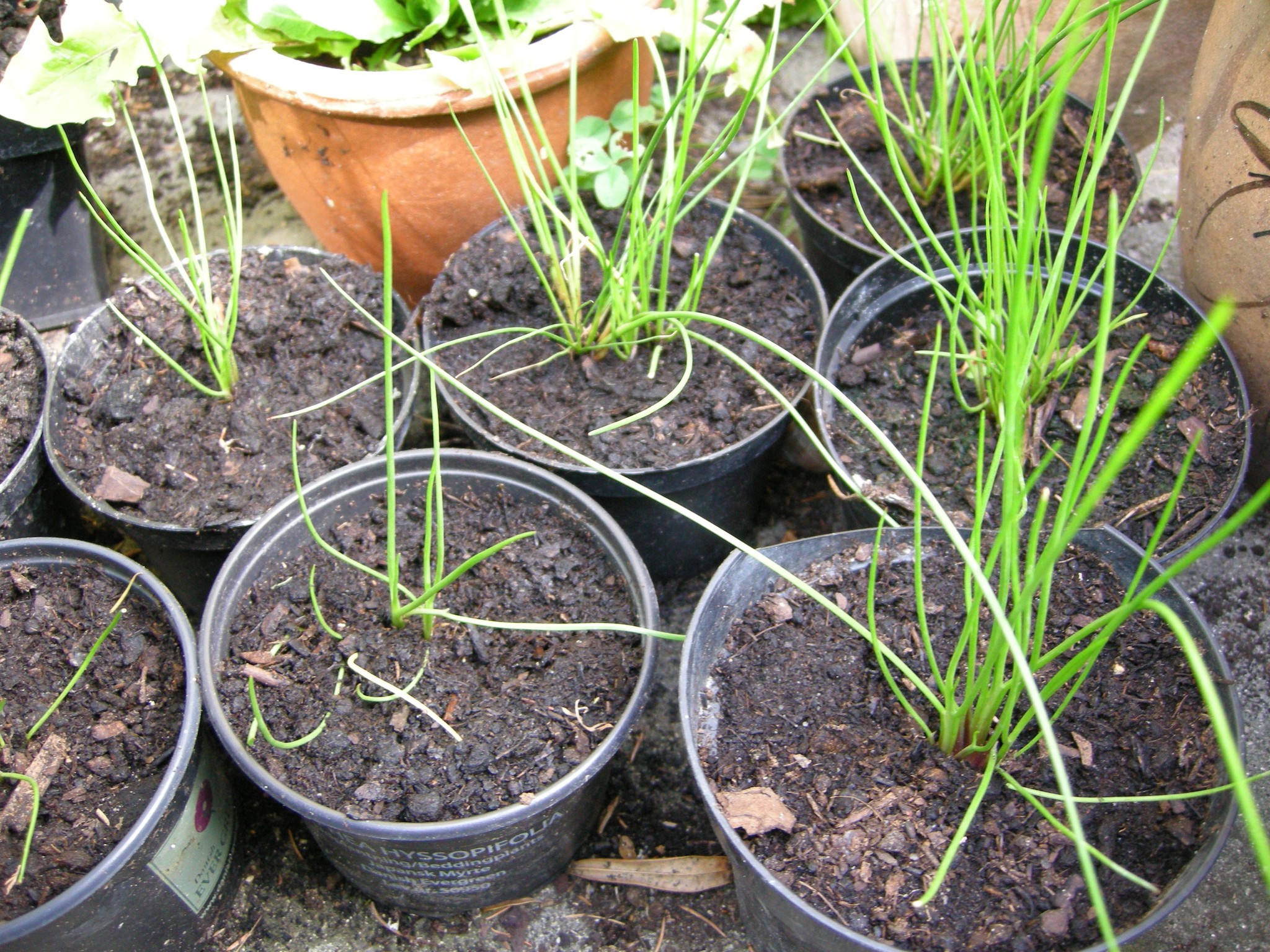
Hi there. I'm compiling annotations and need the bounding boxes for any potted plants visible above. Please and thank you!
[0,0,1270,952]
